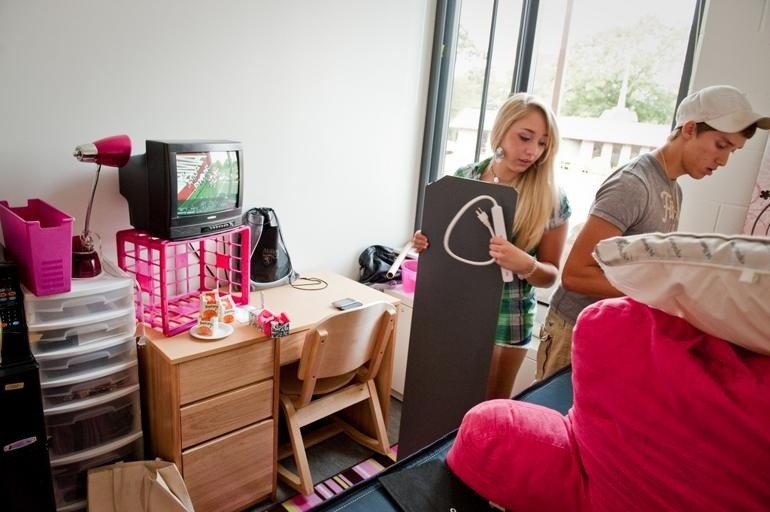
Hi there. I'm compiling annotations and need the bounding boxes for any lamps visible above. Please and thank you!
[72,134,131,278]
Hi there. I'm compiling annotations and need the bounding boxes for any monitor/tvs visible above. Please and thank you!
[116,140,244,239]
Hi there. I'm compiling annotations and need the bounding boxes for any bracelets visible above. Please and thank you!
[517,257,538,280]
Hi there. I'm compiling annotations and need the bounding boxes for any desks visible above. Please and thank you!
[135,267,401,512]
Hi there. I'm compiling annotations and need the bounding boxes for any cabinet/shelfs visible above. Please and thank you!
[20,269,144,512]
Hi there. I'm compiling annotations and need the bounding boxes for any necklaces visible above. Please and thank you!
[656,149,678,232]
[490,165,518,183]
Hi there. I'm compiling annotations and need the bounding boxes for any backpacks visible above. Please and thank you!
[237,206,329,293]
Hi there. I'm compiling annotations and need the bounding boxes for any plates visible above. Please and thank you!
[189,321,235,340]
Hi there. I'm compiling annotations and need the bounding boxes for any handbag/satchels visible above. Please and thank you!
[84,455,196,512]
[357,243,420,285]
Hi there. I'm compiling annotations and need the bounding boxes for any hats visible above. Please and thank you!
[674,84,770,134]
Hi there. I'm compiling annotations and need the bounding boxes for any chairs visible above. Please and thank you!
[277,302,396,495]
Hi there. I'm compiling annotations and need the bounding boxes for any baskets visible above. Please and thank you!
[115,222,253,340]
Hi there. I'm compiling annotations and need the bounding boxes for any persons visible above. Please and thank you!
[533,85,770,383]
[410,92,572,399]
